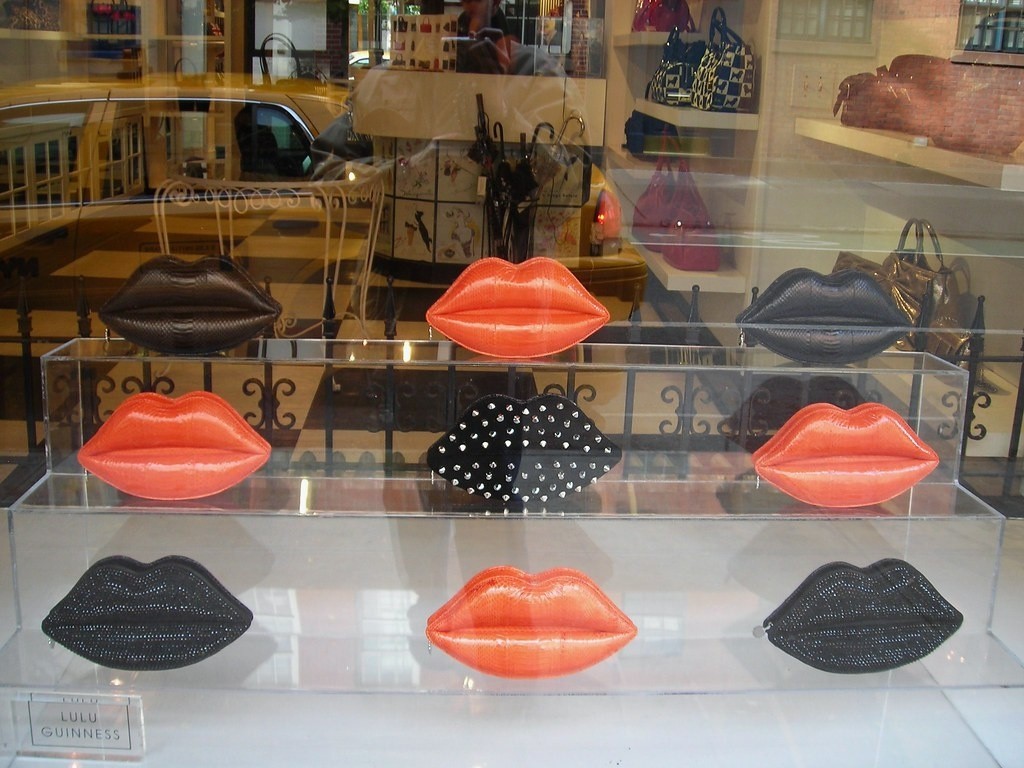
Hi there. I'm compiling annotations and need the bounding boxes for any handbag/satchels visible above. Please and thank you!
[233,32,338,180]
[425,566,636,682]
[832,64,943,137]
[77,392,272,502]
[761,558,963,676]
[98,256,282,356]
[392,15,457,72]
[41,554,254,671]
[735,217,983,388]
[630,0,697,33]
[427,258,612,360]
[621,109,681,163]
[751,403,939,508]
[644,26,709,106]
[631,131,720,271]
[426,394,622,505]
[690,6,757,113]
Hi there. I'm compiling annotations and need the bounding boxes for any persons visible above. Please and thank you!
[308,0,594,717]
[451,0,518,75]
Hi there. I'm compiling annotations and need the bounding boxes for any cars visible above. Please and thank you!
[0,74,355,189]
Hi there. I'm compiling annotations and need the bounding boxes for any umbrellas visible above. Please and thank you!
[470,91,585,265]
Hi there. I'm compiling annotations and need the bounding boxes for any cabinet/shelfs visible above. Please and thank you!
[795,118,1023,457]
[608,31,758,293]
[0,0,243,189]
